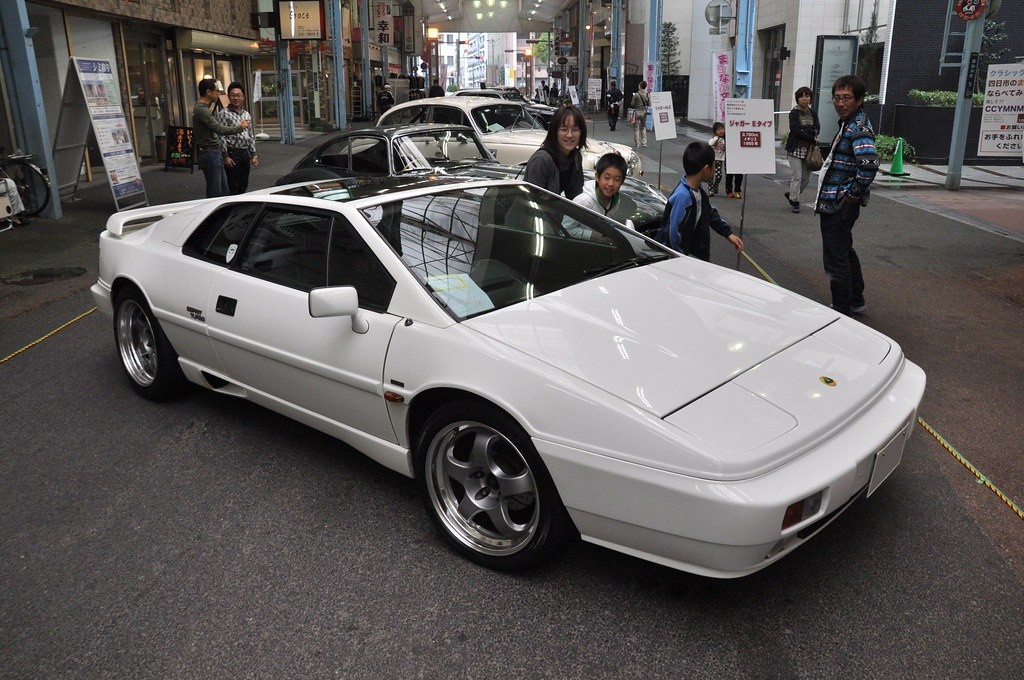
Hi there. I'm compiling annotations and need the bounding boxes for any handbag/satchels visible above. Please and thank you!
[646,108,651,114]
[806,129,822,171]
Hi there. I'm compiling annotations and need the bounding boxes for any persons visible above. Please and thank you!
[814,75,879,315]
[726,174,743,199]
[541,80,549,96]
[631,81,650,148]
[709,122,725,196]
[606,81,622,131]
[550,83,558,97]
[523,106,588,201]
[665,141,744,262]
[428,79,444,97]
[559,153,637,245]
[784,86,821,213]
[193,79,250,199]
[214,82,259,196]
[379,85,394,116]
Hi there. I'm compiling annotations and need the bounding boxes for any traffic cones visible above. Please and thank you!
[881,136,911,177]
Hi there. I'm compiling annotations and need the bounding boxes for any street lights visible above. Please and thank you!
[525,49,531,97]
[426,27,439,89]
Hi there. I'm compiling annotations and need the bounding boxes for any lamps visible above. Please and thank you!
[780,46,790,61]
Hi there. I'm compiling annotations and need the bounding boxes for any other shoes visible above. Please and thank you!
[734,192,740,198]
[709,191,714,196]
[727,192,734,198]
[792,205,800,213]
[784,192,794,206]
[831,294,866,313]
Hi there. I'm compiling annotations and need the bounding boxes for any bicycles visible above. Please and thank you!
[0,154,52,218]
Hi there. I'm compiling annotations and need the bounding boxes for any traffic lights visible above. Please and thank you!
[475,56,483,59]
[458,40,469,44]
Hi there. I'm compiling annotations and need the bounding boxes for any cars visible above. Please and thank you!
[339,95,643,180]
[92,177,927,579]
[274,124,669,244]
[446,81,560,131]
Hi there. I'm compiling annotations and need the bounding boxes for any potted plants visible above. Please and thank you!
[893,88,1023,166]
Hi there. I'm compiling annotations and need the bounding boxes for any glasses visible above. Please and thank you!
[831,96,856,103]
[230,93,244,98]
